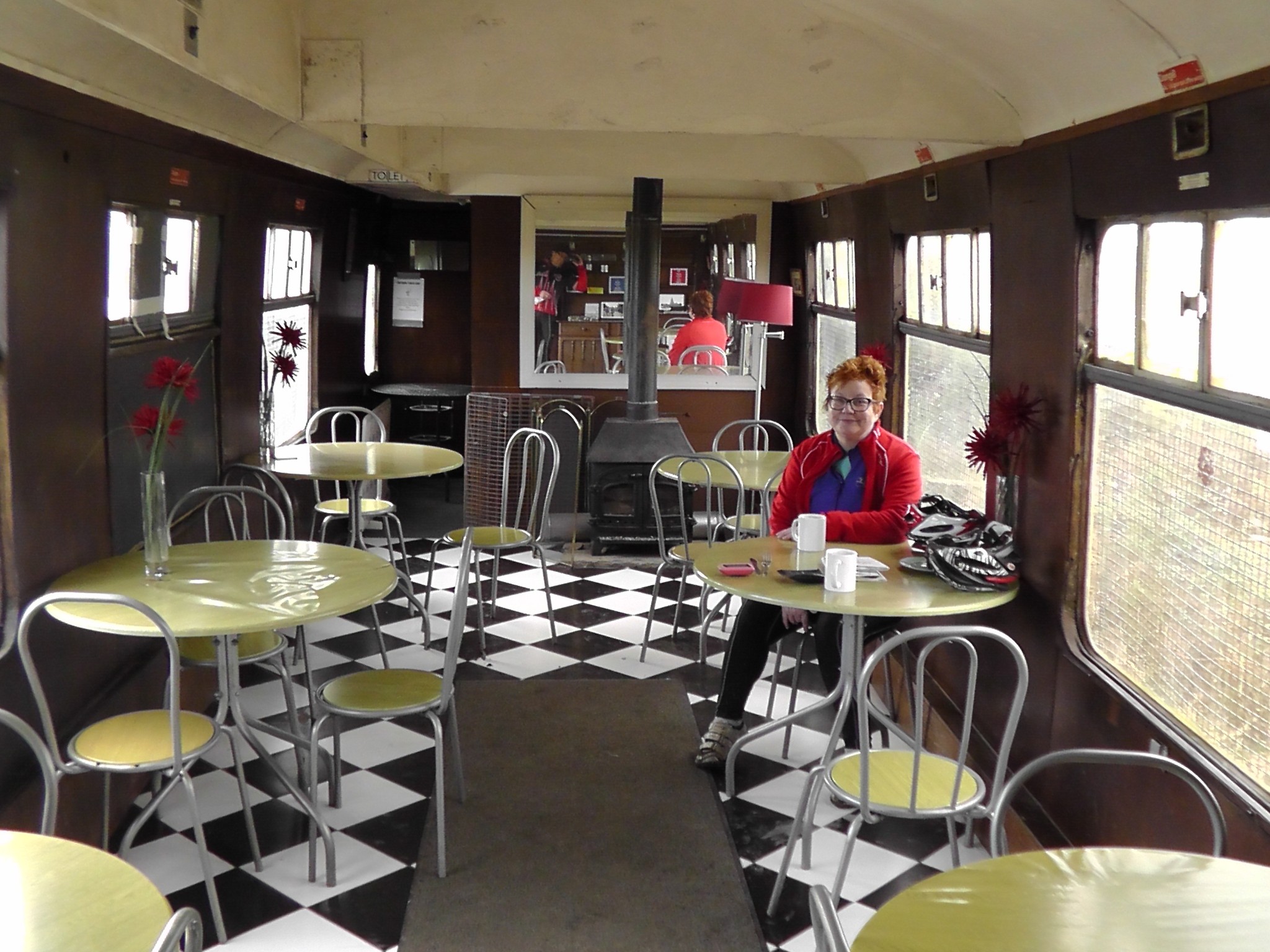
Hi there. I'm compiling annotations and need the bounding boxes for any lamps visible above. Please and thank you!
[715,276,754,352]
[735,281,793,448]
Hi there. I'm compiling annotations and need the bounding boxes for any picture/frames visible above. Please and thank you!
[669,268,688,286]
[601,301,624,319]
[609,276,625,293]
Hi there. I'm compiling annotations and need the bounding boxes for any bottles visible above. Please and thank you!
[585,254,610,274]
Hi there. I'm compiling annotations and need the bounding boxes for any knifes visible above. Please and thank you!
[750,557,761,575]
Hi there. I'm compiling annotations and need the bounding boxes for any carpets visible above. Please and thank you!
[113,538,990,952]
[396,680,769,952]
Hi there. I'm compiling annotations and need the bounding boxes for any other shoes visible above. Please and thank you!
[831,794,855,809]
[695,719,747,768]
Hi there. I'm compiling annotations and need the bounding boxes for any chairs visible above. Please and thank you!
[535,316,727,373]
[0,409,1270,952]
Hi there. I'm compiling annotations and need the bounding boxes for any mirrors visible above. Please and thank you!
[520,194,772,389]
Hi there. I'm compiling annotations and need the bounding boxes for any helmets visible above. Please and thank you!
[905,492,1025,592]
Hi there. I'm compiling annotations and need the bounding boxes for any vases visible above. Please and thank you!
[995,475,1028,563]
[139,471,171,580]
[259,391,276,460]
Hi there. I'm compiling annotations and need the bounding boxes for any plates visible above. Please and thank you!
[777,568,825,583]
[822,556,890,571]
[899,556,934,572]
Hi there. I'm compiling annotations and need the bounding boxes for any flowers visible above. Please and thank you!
[964,350,1042,476]
[131,338,212,461]
[260,320,308,390]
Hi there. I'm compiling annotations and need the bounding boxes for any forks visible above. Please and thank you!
[761,553,772,576]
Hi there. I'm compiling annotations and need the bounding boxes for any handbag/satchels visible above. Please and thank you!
[535,285,556,314]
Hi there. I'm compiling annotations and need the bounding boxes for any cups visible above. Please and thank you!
[823,548,858,592]
[791,514,827,552]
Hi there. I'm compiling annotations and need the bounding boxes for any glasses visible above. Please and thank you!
[828,394,875,412]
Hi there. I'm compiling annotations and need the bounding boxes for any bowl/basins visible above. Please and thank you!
[718,563,755,576]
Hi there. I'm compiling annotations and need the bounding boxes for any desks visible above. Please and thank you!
[43,539,399,887]
[244,442,463,650]
[693,537,1017,796]
[372,383,471,397]
[613,347,667,361]
[658,450,792,492]
[658,364,740,373]
[658,328,679,336]
[606,336,622,344]
[848,847,1270,952]
[0,830,182,952]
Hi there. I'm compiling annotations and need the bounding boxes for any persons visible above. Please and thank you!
[667,290,728,369]
[534,244,588,373]
[695,354,923,810]
